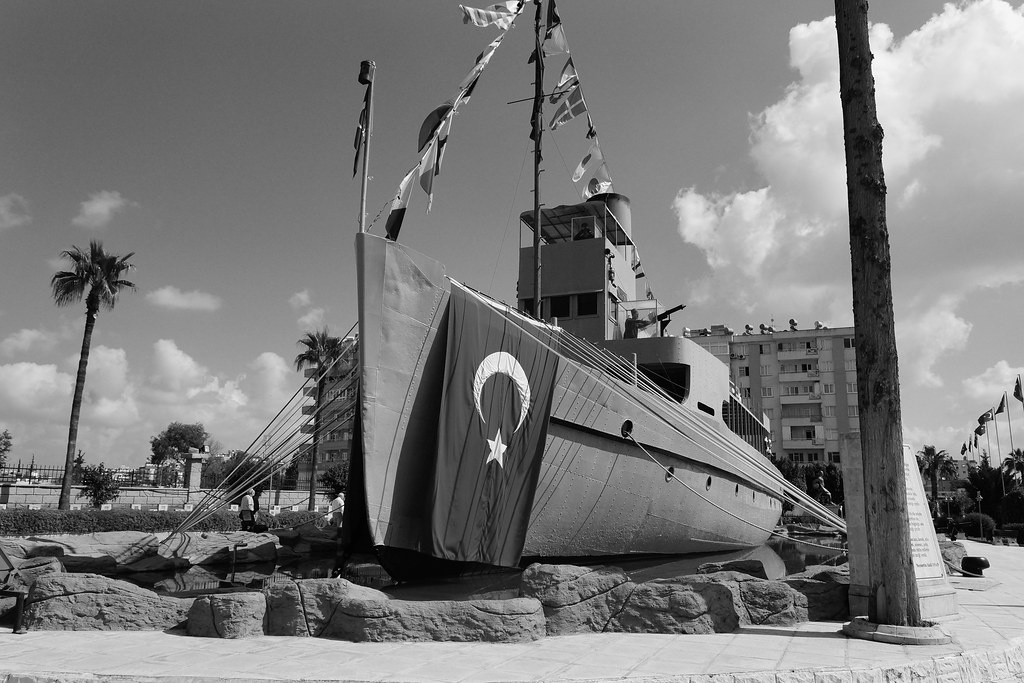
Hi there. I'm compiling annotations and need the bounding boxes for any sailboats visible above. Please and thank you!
[356,0,787,583]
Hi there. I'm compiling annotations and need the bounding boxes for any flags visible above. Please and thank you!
[549,57,578,103]
[418,140,436,213]
[458,0,521,30]
[571,138,603,182]
[417,89,467,153]
[525,24,567,64]
[384,169,415,242]
[581,164,612,198]
[434,115,452,175]
[352,107,366,178]
[430,279,561,569]
[450,32,505,104]
[961,378,1024,456]
[548,87,586,131]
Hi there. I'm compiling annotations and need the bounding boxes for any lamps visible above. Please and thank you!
[199,445,210,454]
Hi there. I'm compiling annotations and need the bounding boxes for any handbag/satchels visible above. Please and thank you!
[242,510,252,521]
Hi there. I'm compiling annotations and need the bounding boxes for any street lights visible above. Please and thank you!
[944,497,953,518]
[976,495,983,541]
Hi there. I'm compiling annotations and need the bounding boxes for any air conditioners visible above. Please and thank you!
[730,352,737,358]
[738,353,746,359]
[345,412,353,419]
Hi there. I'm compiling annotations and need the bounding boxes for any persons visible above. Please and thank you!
[573,223,594,242]
[623,308,650,340]
[331,492,346,529]
[240,490,259,533]
